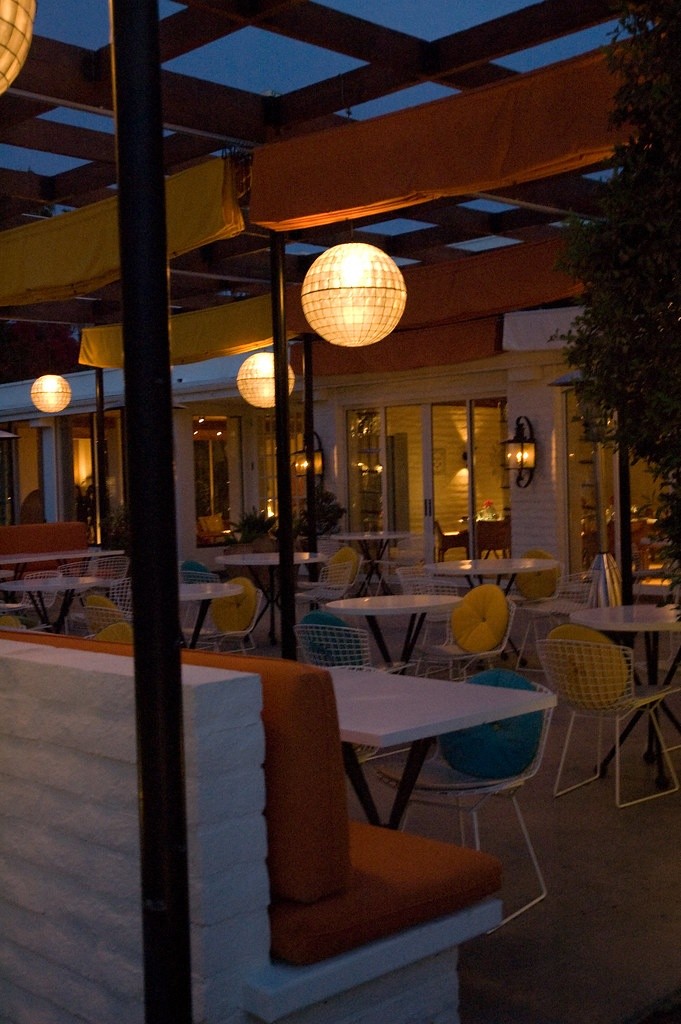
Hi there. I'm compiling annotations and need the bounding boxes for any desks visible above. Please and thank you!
[0,577,115,631]
[568,604,681,790]
[39,548,125,607]
[330,531,405,597]
[459,518,511,559]
[423,559,559,668]
[321,666,560,830]
[0,553,49,618]
[325,593,464,675]
[214,551,331,646]
[179,583,244,648]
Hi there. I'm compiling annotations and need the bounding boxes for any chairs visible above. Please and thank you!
[21,518,681,935]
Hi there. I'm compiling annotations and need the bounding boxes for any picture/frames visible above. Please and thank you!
[432,448,446,475]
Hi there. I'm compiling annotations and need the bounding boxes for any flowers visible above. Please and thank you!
[483,500,496,513]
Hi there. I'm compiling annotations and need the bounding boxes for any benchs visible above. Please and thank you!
[0,522,89,582]
[0,631,507,1024]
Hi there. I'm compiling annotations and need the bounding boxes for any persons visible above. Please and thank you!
[74,483,96,542]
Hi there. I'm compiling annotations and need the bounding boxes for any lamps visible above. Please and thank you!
[292,430,327,488]
[497,414,538,488]
[300,217,408,348]
[463,451,468,468]
[236,347,295,409]
[30,374,72,413]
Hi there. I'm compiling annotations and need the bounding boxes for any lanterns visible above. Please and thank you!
[301,242,406,351]
[30,375,72,412]
[236,352,295,408]
